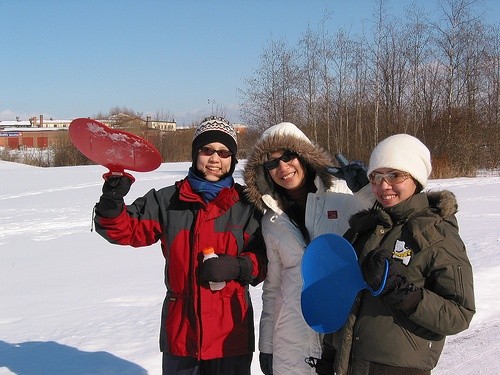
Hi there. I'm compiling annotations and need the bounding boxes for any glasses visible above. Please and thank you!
[263,149,298,171]
[370,169,414,186]
[195,147,234,158]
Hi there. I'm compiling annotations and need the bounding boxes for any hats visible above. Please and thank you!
[189,116,238,159]
[366,134,432,187]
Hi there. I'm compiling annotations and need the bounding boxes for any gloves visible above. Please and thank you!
[258,351,273,375]
[200,254,240,282]
[326,153,369,193]
[316,345,336,375]
[102,176,132,199]
[360,248,410,305]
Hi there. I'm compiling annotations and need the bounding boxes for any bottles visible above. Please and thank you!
[201,247,227,291]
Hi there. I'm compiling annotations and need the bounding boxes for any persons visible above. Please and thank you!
[241,121,378,375]
[316,133,476,375]
[94,116,267,375]
